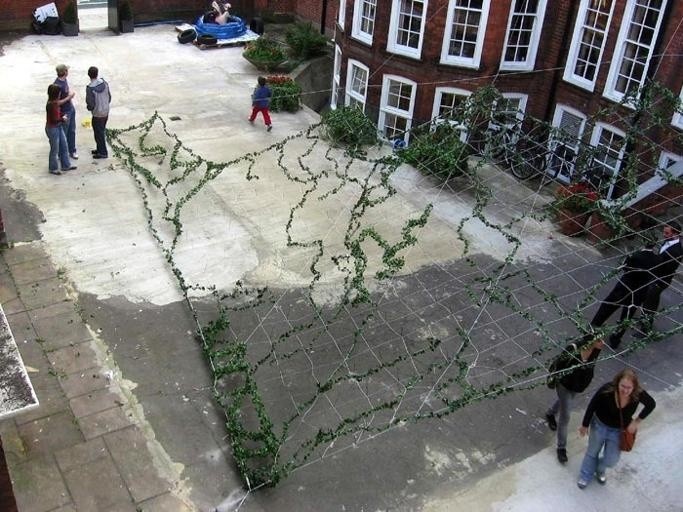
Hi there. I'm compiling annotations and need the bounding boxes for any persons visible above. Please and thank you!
[43,83,77,174]
[544,330,613,462]
[629,222,683,338]
[576,367,655,489]
[50,64,78,160]
[248,77,272,132]
[591,232,662,350]
[86,67,110,160]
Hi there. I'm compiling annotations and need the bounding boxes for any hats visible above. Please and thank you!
[56,64,70,75]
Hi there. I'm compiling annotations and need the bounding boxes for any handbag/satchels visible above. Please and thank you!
[619,427,638,452]
[546,360,558,390]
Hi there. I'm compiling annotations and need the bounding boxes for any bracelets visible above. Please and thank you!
[635,418,642,424]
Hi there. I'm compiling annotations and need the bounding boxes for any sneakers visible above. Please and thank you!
[546,414,557,431]
[70,152,79,159]
[577,476,588,489]
[62,165,78,171]
[91,150,98,154]
[596,472,607,483]
[51,170,62,175]
[93,153,107,159]
[557,447,568,462]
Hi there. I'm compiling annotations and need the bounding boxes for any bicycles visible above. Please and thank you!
[476,117,547,178]
